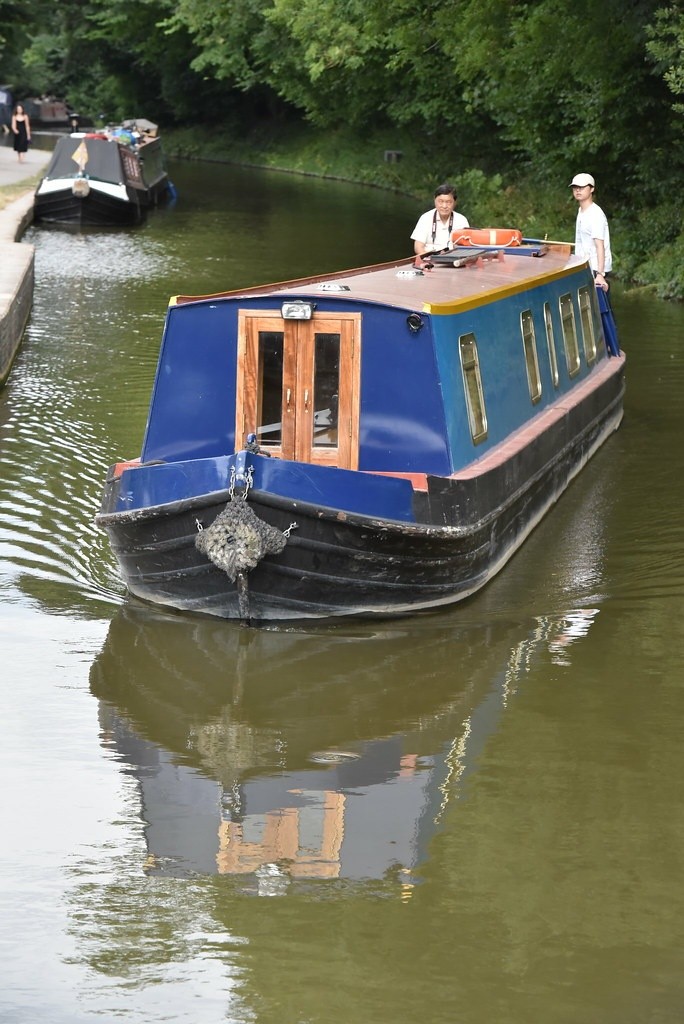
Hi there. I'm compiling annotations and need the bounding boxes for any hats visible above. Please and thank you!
[569,173,595,188]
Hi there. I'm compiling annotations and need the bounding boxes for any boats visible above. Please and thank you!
[90,515,574,898]
[91,225,628,619]
[33,117,177,235]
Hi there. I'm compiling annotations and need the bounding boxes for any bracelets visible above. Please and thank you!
[597,272,606,277]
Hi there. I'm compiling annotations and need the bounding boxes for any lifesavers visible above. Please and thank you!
[451,227,523,246]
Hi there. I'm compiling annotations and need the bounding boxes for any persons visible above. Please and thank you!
[410,185,469,256]
[569,172,612,292]
[0,105,31,162]
